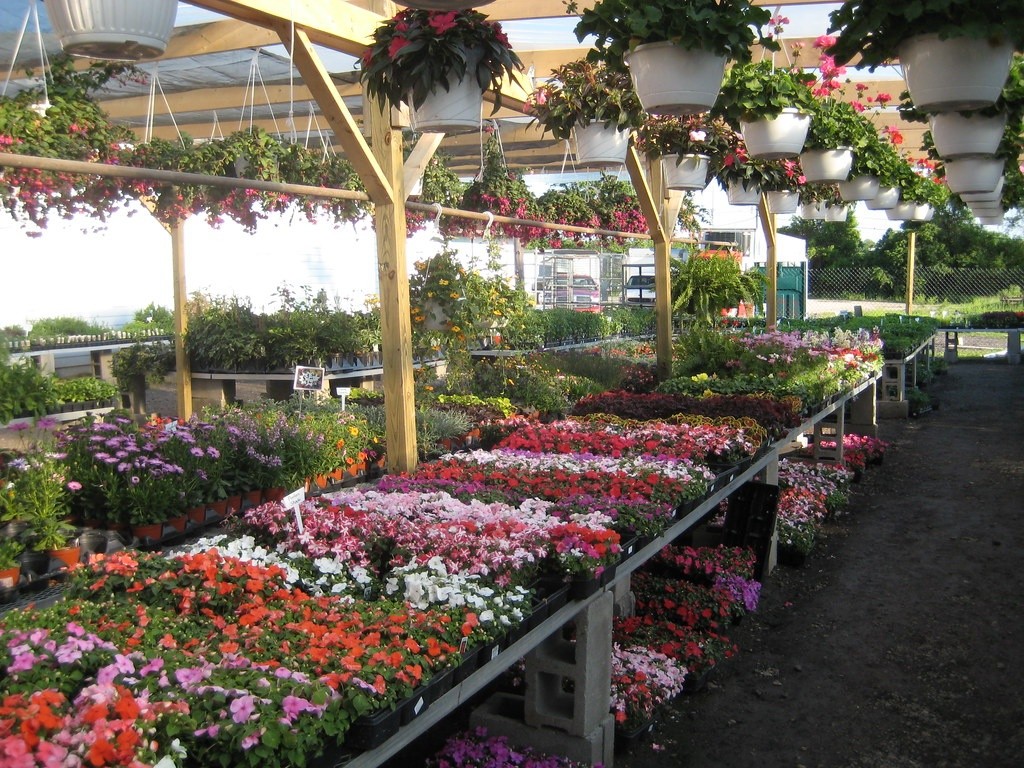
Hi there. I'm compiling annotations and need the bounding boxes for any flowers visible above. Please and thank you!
[1,8,653,253]
[521,16,944,204]
[1,248,1024,768]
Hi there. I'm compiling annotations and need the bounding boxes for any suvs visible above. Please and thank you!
[620,273,655,308]
[543,274,600,313]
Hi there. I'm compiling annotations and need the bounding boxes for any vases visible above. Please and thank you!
[0,567,20,587]
[740,109,812,160]
[420,297,451,331]
[799,145,853,182]
[46,547,81,567]
[44,0,178,59]
[865,185,899,209]
[838,174,880,202]
[728,534,772,584]
[914,202,929,222]
[767,190,800,214]
[726,480,781,535]
[726,176,763,206]
[885,201,915,220]
[570,117,632,166]
[661,153,711,190]
[404,47,482,130]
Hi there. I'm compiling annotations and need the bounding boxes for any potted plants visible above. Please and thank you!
[561,0,772,117]
[826,1,1024,225]
